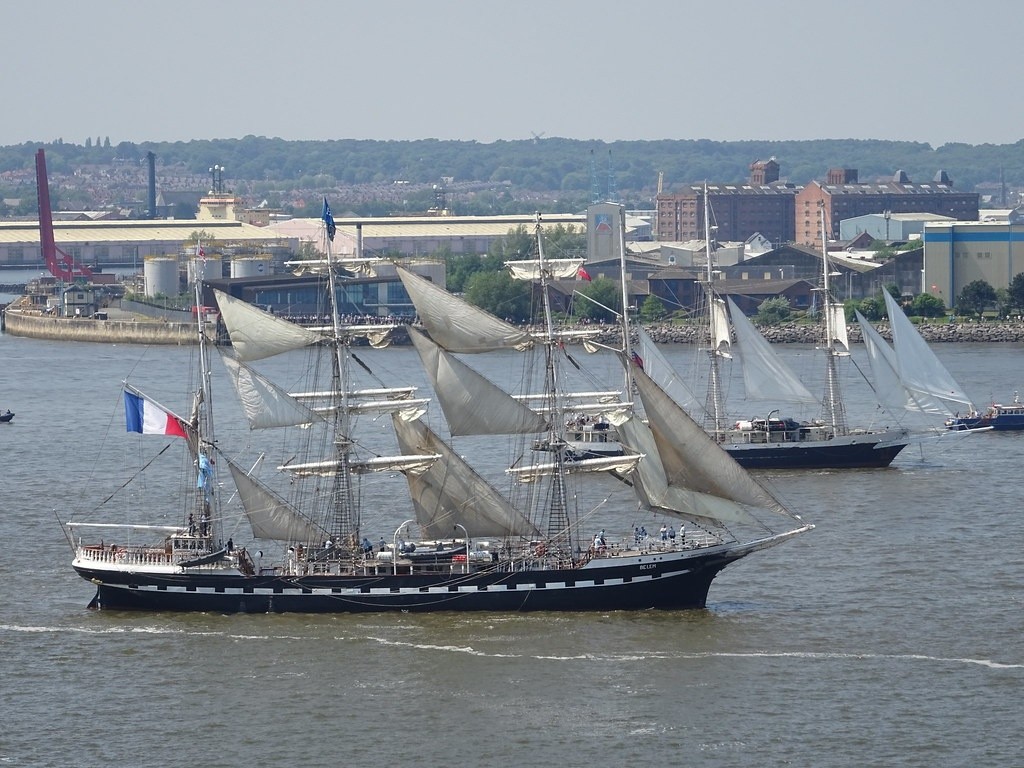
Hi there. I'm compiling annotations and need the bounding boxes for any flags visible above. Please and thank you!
[321,196,335,242]
[124,389,188,438]
[199,244,205,263]
[579,264,591,282]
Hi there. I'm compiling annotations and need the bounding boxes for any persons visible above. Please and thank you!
[660,524,667,545]
[851,314,1024,323]
[668,526,675,544]
[379,537,387,552]
[227,538,233,556]
[363,538,374,559]
[600,528,607,549]
[563,412,605,430]
[680,523,685,545]
[277,313,416,326]
[594,535,602,549]
[504,315,710,326]
[634,528,640,544]
[436,543,444,551]
[188,513,197,535]
[640,526,647,539]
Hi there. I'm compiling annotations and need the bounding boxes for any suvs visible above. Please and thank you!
[89,311,107,320]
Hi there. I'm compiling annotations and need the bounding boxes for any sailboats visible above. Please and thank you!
[54,195,818,614]
[503,181,918,475]
[843,284,1024,443]
